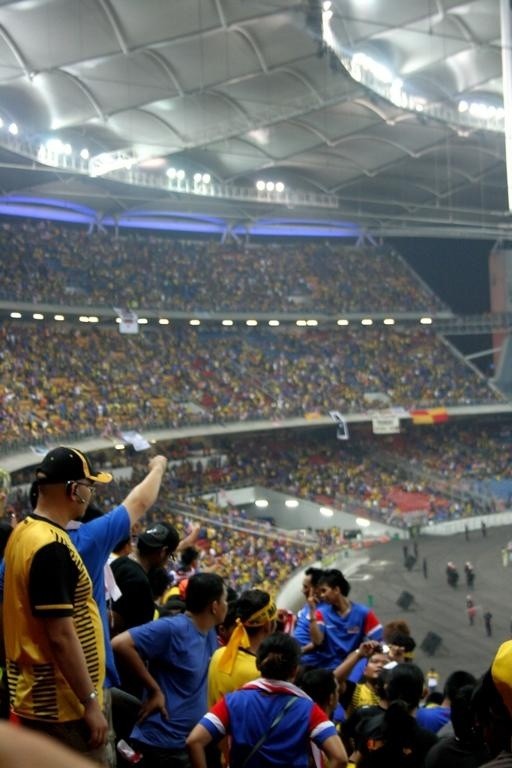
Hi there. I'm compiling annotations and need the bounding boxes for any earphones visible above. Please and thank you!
[66,481,78,497]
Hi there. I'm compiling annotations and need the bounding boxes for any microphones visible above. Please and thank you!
[74,493,85,503]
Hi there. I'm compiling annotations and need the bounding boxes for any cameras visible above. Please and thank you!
[375,644,391,654]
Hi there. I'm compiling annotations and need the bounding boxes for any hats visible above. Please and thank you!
[139,521,178,550]
[37,446,113,486]
